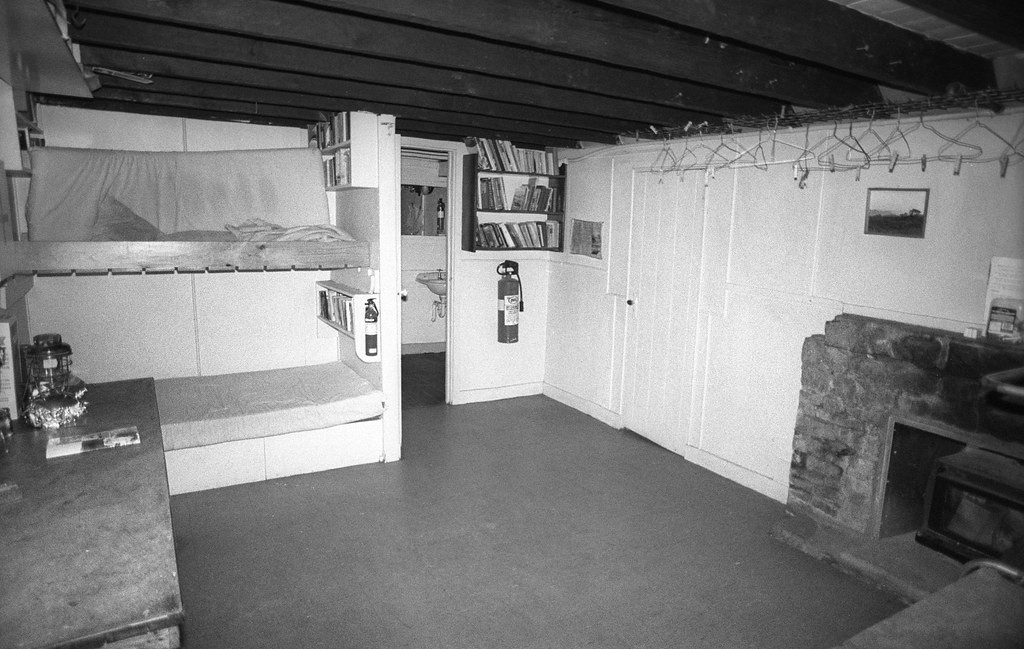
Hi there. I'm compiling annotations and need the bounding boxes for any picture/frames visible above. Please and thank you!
[865,188,931,238]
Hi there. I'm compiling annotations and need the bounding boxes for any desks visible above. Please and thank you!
[0,377,188,648]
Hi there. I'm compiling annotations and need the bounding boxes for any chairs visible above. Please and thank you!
[824,559,1024,649]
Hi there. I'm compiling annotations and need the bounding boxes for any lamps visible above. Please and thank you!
[24,333,75,398]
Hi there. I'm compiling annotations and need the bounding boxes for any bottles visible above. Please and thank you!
[0,408,13,437]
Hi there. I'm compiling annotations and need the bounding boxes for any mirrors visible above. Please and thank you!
[401,184,448,237]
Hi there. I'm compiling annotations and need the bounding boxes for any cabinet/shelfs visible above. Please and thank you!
[308,111,378,190]
[0,0,94,101]
[1,84,46,175]
[462,152,568,255]
[314,280,381,364]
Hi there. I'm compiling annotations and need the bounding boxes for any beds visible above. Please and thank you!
[0,113,401,497]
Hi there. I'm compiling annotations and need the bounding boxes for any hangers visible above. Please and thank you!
[643,94,1024,178]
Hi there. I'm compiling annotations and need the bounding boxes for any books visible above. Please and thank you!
[320,289,353,333]
[477,216,559,247]
[46,426,141,459]
[480,178,557,212]
[463,137,560,175]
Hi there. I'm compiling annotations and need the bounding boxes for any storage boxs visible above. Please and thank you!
[0,316,24,421]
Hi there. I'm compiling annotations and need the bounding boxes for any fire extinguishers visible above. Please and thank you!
[496,260,524,343]
[365,298,379,356]
[437,198,445,231]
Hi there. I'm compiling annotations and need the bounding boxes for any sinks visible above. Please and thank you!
[415,271,448,295]
